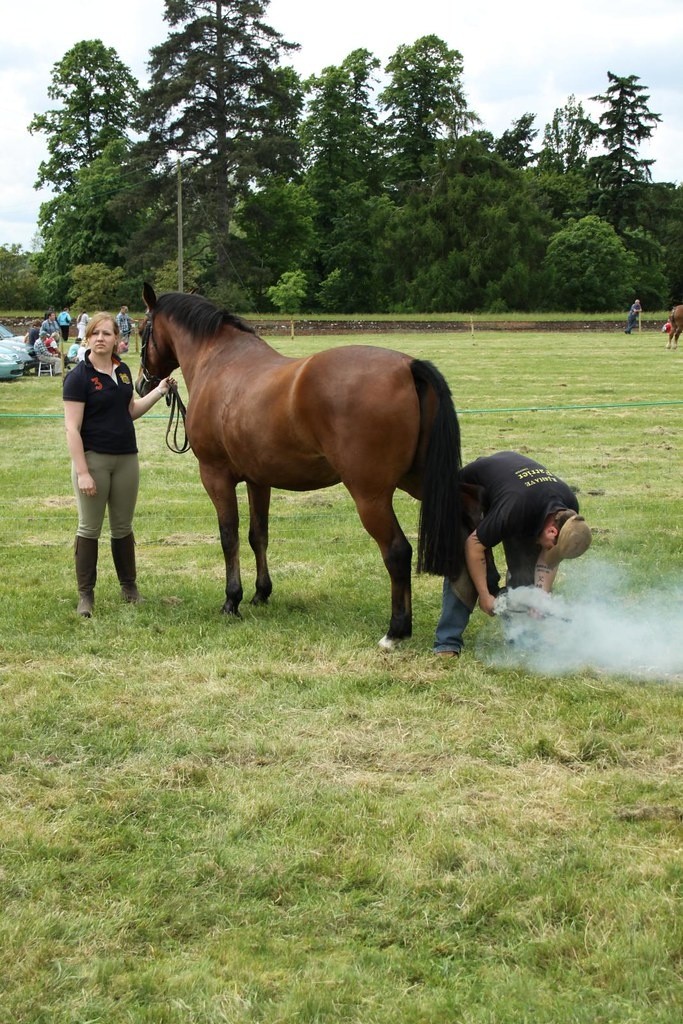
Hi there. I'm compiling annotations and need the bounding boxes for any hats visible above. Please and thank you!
[544,515,592,569]
[39,331,50,337]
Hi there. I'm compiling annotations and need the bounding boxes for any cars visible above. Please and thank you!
[0,325,39,380]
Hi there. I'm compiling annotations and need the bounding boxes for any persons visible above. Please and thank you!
[24,320,41,346]
[434,450,593,662]
[45,331,62,358]
[57,307,72,341]
[64,311,173,616]
[67,338,90,364]
[34,332,63,376]
[662,318,672,333]
[39,313,62,342]
[44,307,57,323]
[76,310,92,338]
[116,304,139,351]
[624,300,642,334]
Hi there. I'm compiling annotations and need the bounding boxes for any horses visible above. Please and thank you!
[666,305,683,349]
[135,283,462,650]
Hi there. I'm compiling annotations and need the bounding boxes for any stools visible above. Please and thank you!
[37,361,53,376]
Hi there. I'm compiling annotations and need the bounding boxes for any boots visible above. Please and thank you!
[111,531,140,603]
[74,536,99,617]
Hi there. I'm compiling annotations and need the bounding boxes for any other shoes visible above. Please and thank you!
[438,650,459,663]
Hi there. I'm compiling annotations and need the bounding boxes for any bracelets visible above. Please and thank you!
[157,387,162,394]
